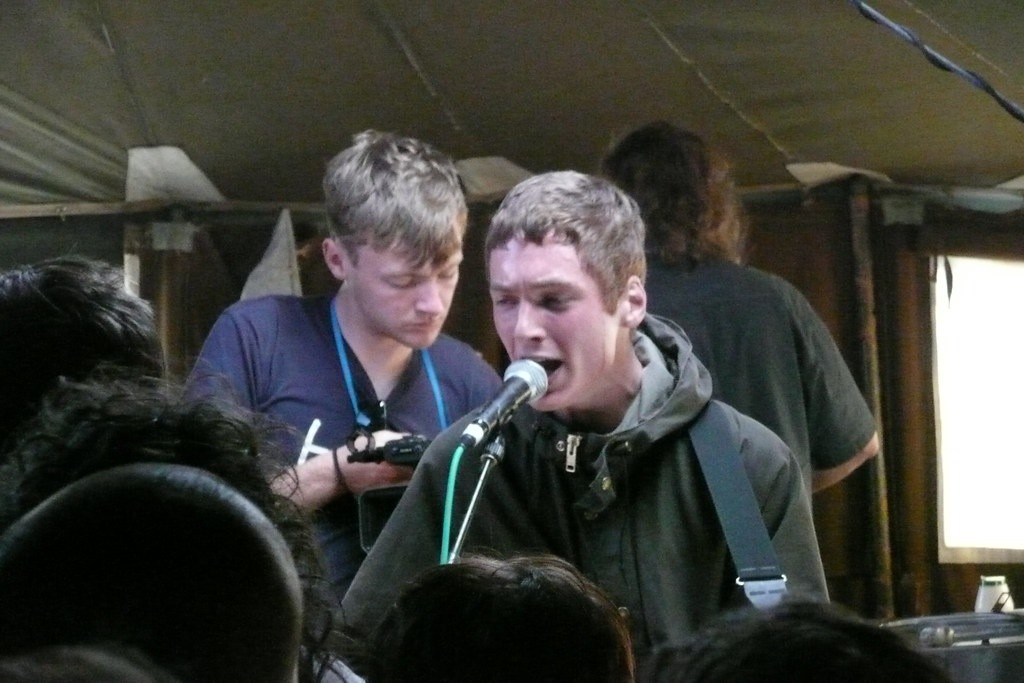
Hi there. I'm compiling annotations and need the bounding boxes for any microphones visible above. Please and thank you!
[457,357,548,452]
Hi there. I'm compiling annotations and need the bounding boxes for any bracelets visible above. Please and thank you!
[333,446,350,497]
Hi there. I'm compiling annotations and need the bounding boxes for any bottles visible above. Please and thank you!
[974,576,1015,613]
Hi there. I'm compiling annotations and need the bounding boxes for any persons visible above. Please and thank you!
[333,167,831,683]
[0,251,960,683]
[176,126,504,610]
[595,119,881,515]
